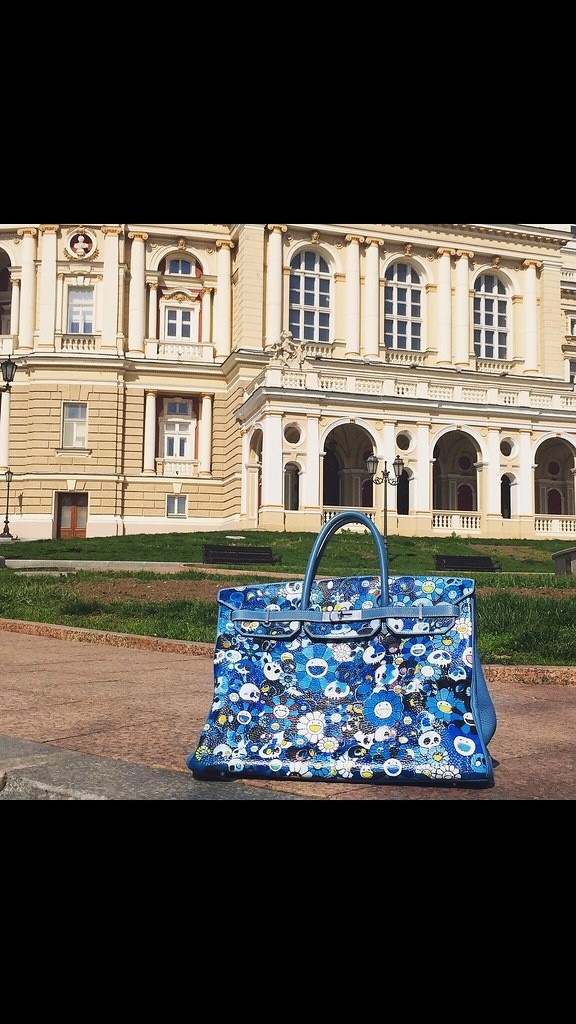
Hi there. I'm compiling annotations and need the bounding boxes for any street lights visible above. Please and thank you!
[364,452,405,562]
[0,467,14,538]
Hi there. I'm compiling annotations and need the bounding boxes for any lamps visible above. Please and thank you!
[499,371,509,377]
[410,362,419,370]
[314,354,323,360]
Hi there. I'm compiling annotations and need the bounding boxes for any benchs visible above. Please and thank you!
[203,543,279,566]
[433,554,503,573]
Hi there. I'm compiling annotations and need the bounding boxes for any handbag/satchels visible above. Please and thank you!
[184,512,501,787]
